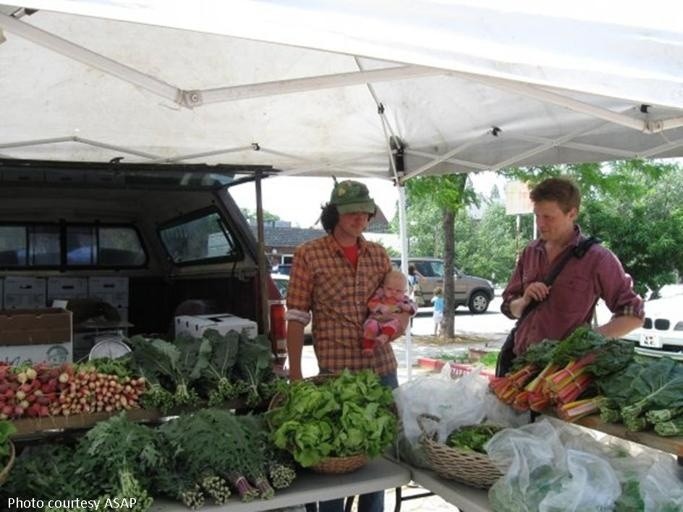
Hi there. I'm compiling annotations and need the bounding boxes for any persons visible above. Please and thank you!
[494,176,646,426]
[430,286,443,336]
[407,262,423,330]
[284,180,411,511]
[360,271,419,360]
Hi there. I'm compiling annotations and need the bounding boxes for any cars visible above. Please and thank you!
[617,294,683,364]
[268,255,495,337]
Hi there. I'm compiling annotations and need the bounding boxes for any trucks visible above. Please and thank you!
[0,154,291,441]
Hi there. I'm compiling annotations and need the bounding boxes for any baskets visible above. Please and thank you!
[416,414,504,491]
[267,371,369,475]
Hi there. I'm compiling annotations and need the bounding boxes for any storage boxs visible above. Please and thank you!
[174,312,259,354]
[0,274,131,369]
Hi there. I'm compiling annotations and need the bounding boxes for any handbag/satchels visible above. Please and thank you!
[495,328,514,377]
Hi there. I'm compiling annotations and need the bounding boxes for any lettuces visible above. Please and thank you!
[444,424,504,452]
[271,367,397,469]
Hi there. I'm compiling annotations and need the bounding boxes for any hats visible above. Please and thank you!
[328,180,375,214]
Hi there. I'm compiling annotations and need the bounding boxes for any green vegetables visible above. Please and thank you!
[7,410,298,511]
